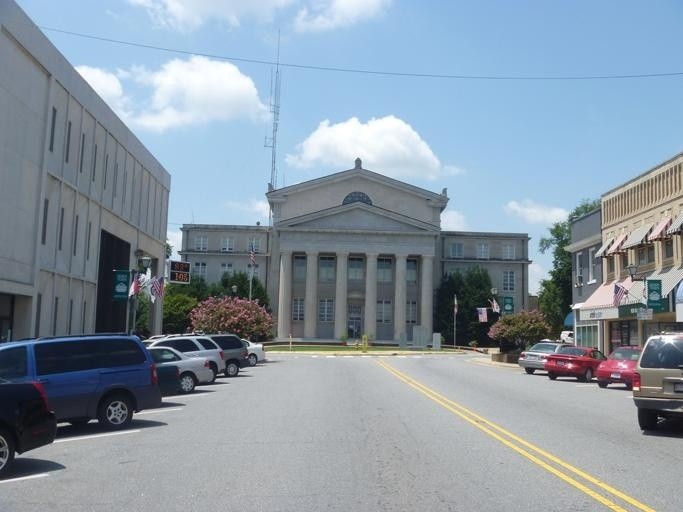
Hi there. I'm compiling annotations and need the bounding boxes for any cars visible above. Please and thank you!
[142,333,267,397]
[0,375,56,478]
[546,347,607,384]
[596,345,644,391]
[518,343,574,376]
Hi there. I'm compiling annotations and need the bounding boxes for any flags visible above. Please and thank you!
[475,307,487,324]
[487,298,492,309]
[128,272,163,305]
[492,298,499,313]
[453,296,458,315]
[612,284,624,308]
[249,243,256,270]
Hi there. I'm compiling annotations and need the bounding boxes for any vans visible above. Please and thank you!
[0,333,164,430]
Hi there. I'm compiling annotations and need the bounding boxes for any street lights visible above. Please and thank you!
[624,262,647,345]
[231,282,239,297]
[490,288,498,300]
[125,254,152,336]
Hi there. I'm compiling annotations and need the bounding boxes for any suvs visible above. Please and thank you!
[633,330,682,434]
